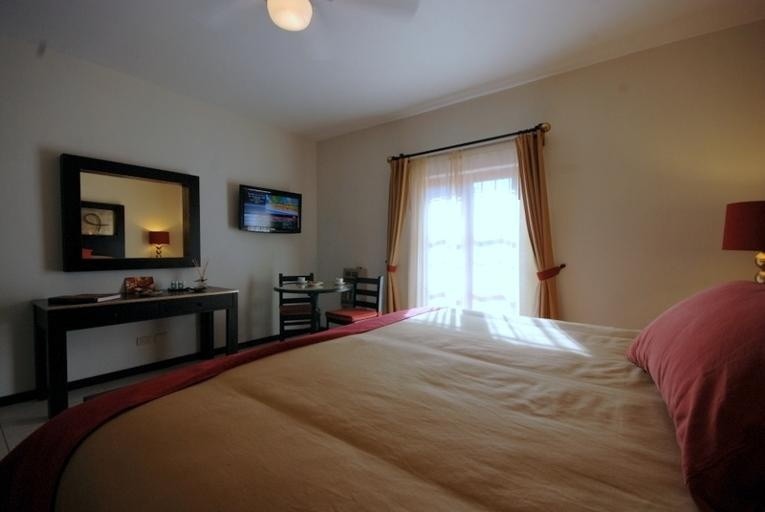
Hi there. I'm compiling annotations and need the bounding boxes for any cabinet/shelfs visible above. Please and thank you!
[29,286,239,421]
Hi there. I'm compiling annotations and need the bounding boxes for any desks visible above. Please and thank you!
[274,280,356,330]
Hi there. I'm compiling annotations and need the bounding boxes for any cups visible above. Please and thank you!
[178,279,183,288]
[297,277,305,282]
[170,280,175,288]
[336,278,343,283]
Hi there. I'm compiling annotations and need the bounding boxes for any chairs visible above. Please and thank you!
[277,272,321,340]
[326,274,384,329]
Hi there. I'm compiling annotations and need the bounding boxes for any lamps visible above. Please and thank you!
[147,230,171,258]
[265,0,314,33]
[721,198,765,283]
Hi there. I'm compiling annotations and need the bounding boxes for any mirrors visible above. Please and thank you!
[60,153,202,271]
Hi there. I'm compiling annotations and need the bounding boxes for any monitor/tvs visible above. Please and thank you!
[239,184,302,233]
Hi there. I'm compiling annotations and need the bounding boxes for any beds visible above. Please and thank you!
[0,303,698,512]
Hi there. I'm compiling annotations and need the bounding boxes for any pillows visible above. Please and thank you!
[622,279,764,508]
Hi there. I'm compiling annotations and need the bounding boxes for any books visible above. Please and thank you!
[48,293,121,303]
